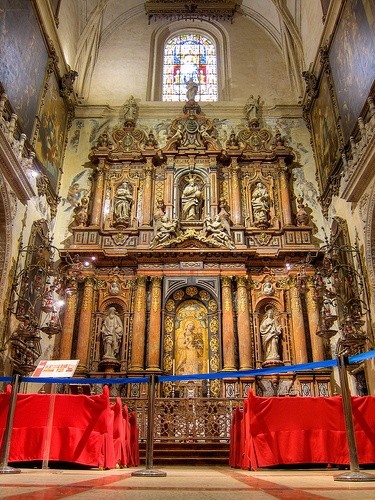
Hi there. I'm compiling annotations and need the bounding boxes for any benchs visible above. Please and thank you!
[0,384,141,471]
[229,387,375,472]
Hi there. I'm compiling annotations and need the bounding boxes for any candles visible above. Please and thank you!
[207,359,209,380]
[173,359,177,377]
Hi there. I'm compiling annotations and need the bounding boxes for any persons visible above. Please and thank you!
[99,306,123,359]
[185,78,198,100]
[183,178,202,220]
[245,94,261,121]
[115,181,132,218]
[260,308,282,359]
[251,183,270,221]
[123,96,137,122]
